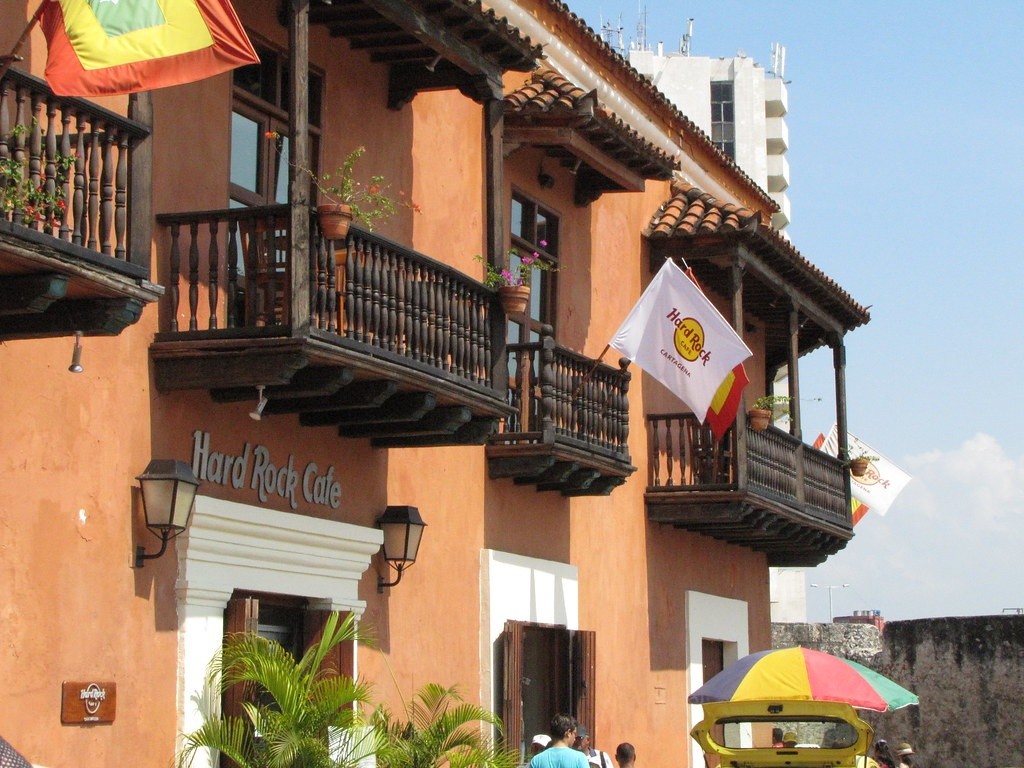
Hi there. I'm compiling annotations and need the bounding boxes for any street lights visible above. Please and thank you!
[810,584,850,623]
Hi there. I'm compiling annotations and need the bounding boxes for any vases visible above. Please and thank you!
[501,286,530,314]
[318,202,354,239]
[747,408,771,430]
[851,458,869,475]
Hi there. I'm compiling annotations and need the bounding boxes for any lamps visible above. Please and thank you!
[539,173,556,190]
[68,331,84,375]
[769,297,778,308]
[425,53,443,74]
[817,332,829,343]
[746,324,758,334]
[799,317,809,329]
[571,160,582,175]
[135,459,201,567]
[377,505,429,595]
[248,382,269,420]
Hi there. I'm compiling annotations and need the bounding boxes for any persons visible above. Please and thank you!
[516,712,613,768]
[771,728,798,748]
[874,740,918,768]
[615,742,636,768]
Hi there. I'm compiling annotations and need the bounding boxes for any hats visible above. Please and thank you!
[575,724,589,737]
[531,734,552,749]
[896,742,915,756]
[783,732,798,745]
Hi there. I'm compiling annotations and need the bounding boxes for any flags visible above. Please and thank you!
[34,0,261,96]
[608,256,753,440]
[810,425,911,527]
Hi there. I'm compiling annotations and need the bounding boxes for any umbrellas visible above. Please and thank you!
[687,644,920,713]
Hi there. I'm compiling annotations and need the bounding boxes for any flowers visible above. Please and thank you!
[268,128,422,232]
[474,239,564,287]
[839,440,881,470]
[0,116,82,230]
[749,394,795,422]
